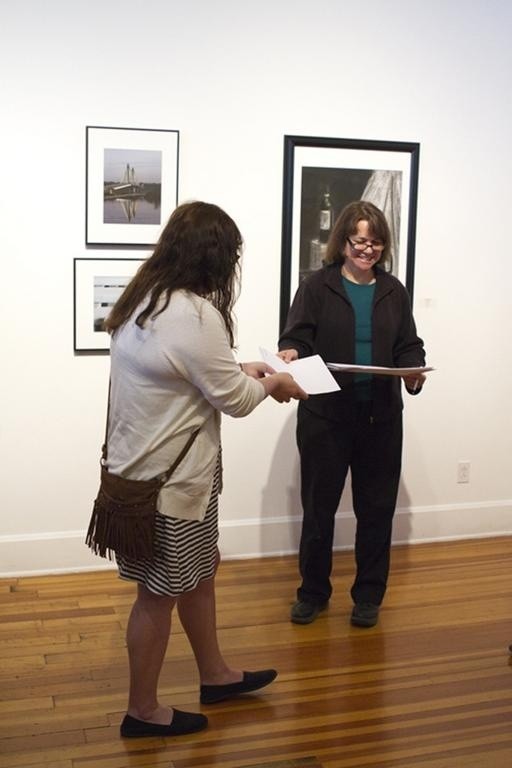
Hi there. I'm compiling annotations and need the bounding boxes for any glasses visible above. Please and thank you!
[346,236,387,252]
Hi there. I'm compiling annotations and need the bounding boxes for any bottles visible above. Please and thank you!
[317,186,335,244]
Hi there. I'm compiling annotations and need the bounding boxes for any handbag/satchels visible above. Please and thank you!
[85,465,166,562]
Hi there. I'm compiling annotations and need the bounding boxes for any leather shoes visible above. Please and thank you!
[290,597,329,624]
[199,669,278,705]
[120,706,208,738]
[350,600,379,628]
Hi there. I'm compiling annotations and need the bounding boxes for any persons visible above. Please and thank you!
[99,199,310,739]
[272,200,428,630]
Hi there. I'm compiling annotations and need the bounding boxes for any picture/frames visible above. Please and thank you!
[84,125,180,245]
[278,134,422,344]
[72,257,154,351]
